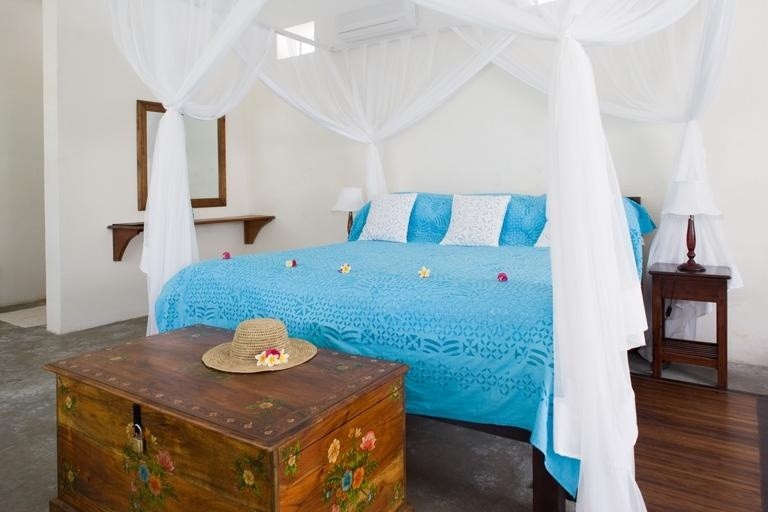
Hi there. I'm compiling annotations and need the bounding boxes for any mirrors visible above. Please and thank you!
[137,98,227,209]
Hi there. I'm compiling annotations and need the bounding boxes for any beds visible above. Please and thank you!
[156,197,643,512]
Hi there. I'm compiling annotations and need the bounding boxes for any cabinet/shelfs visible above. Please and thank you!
[47,322,410,512]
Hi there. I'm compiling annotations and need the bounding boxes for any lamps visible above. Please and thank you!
[668,179,719,273]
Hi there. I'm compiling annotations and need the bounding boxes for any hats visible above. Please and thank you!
[199,318,319,376]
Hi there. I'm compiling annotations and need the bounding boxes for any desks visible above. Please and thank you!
[647,262,732,390]
